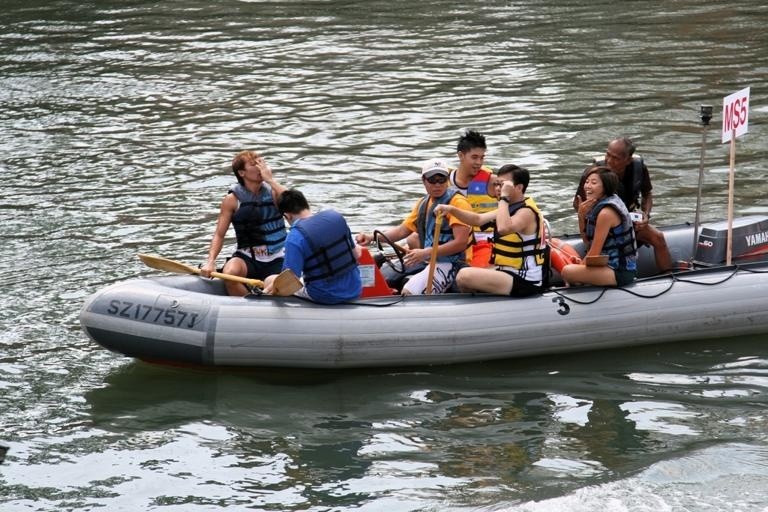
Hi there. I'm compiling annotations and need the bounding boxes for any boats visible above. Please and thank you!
[76,200,768,371]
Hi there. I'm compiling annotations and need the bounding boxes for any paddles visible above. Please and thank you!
[587,255,609,265]
[137,254,263,287]
[350,231,409,252]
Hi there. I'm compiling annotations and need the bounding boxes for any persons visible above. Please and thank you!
[199,152,289,294]
[434,164,553,298]
[447,130,497,267]
[573,135,675,277]
[557,165,637,287]
[260,189,363,303]
[350,157,475,296]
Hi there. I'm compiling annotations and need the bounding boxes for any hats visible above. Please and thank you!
[421,159,448,179]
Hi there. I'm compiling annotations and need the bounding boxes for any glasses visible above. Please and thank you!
[492,182,502,186]
[424,175,449,185]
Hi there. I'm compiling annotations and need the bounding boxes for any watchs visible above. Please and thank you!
[498,194,512,204]
